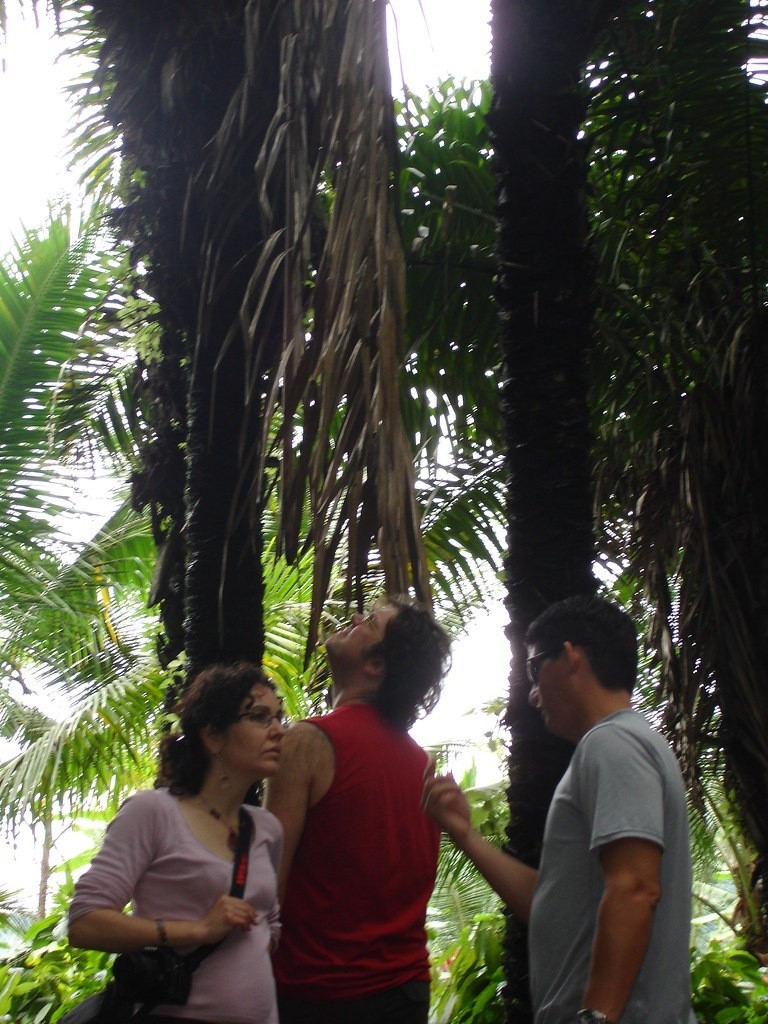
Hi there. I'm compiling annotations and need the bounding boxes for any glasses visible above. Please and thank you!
[361,607,384,644]
[525,641,576,683]
[241,707,287,729]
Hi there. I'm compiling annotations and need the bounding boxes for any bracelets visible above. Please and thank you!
[574,1007,612,1024]
[155,918,169,943]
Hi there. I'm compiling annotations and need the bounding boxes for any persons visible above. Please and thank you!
[68,662,283,1024]
[259,600,454,1023]
[419,594,693,1024]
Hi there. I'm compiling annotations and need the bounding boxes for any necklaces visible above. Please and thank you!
[196,790,238,851]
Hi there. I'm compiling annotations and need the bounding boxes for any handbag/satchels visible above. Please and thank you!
[51,940,192,1024]
